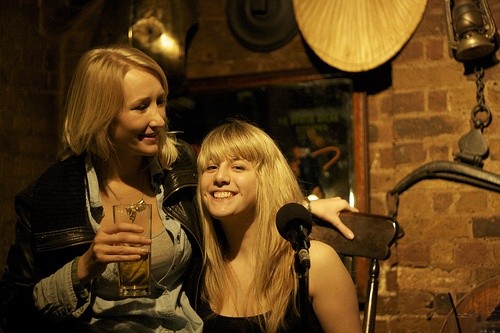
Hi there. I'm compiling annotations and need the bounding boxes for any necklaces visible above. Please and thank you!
[99,170,156,221]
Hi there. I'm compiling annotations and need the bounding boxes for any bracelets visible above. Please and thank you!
[304,197,312,217]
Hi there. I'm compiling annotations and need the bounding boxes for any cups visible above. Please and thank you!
[113,203,152,297]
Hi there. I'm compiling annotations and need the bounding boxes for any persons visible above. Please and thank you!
[193,120,363,333]
[7,46,359,333]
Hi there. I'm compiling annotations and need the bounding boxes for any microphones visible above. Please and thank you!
[275,202,313,268]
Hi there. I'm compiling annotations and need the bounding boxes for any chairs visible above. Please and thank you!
[307,209,405,333]
